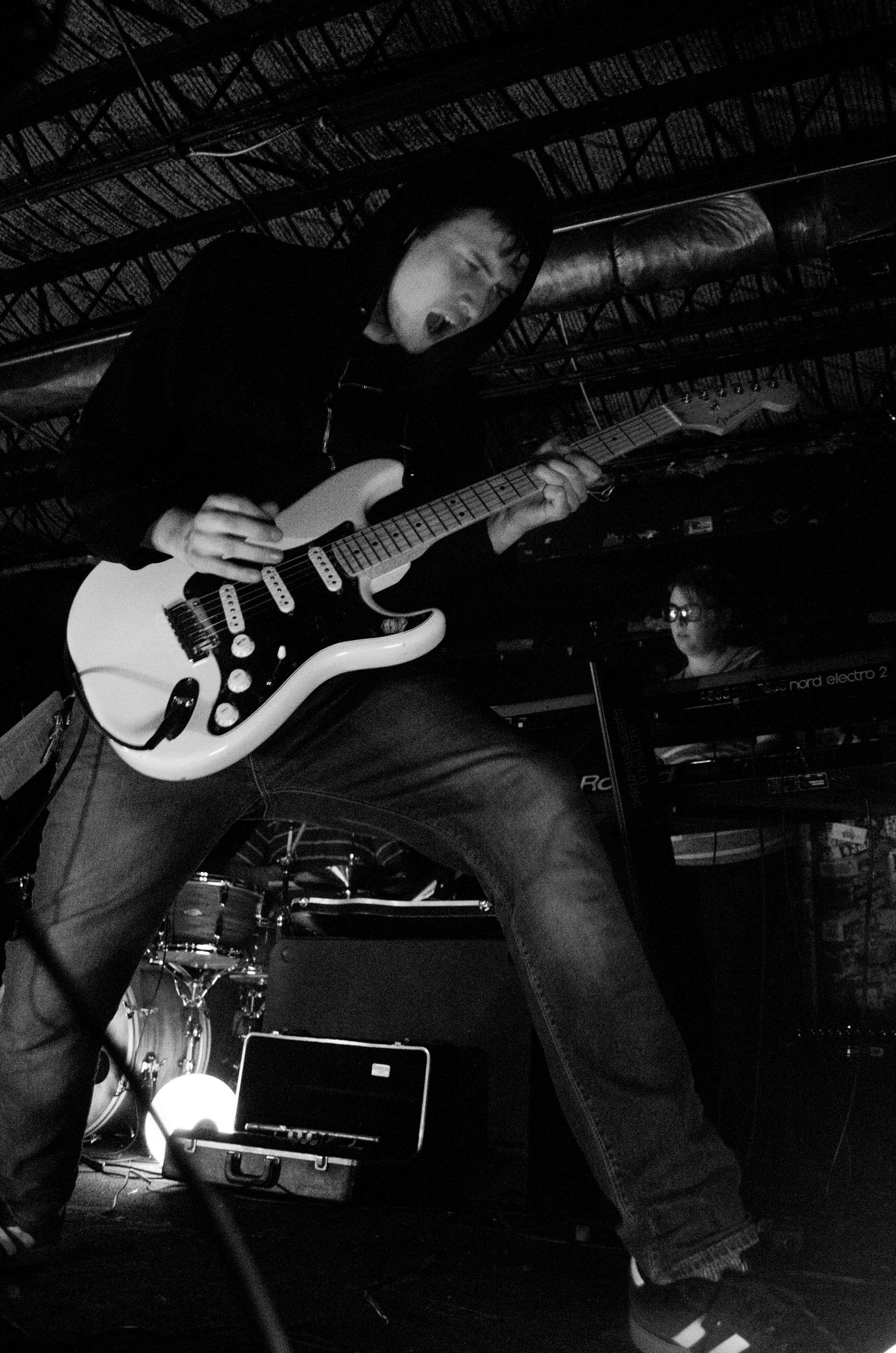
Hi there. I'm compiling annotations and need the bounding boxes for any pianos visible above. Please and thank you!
[483,653,895,872]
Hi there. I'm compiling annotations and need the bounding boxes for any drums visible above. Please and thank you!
[138,866,268,979]
[81,956,215,1141]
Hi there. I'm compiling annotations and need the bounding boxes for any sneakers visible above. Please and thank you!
[0,1215,45,1256]
[627,1243,838,1351]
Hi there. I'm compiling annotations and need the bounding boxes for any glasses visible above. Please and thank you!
[661,603,718,623]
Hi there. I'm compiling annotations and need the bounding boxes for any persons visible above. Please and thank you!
[229,820,419,893]
[633,568,799,872]
[0,155,865,1353]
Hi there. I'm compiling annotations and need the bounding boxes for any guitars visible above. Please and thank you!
[69,367,808,785]
